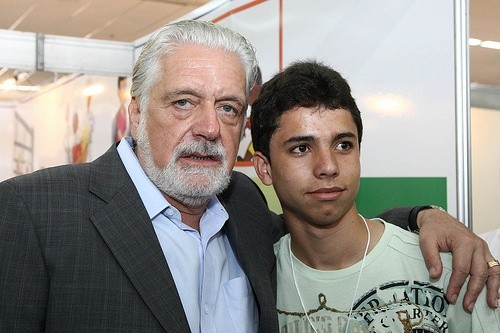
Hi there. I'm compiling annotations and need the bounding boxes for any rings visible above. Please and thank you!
[485,258,499,268]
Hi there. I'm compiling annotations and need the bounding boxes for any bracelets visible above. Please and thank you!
[409,204,446,235]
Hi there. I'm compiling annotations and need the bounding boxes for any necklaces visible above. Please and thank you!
[287,213,372,333]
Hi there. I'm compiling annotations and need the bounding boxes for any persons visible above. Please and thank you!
[0,19,500,333]
[248,62,499,333]
